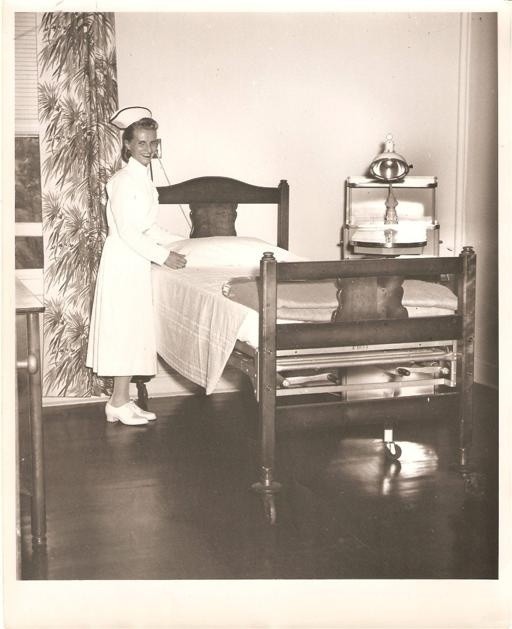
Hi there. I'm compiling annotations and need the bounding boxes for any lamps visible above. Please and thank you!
[366,132,414,247]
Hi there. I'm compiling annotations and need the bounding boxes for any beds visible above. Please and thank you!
[105,175,479,498]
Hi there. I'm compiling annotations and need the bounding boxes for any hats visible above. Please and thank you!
[108,107,152,130]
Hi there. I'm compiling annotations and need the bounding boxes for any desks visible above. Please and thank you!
[16,279,48,552]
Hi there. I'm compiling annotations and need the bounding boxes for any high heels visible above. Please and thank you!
[130,401,156,421]
[105,404,147,425]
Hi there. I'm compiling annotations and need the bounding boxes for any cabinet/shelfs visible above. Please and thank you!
[337,175,441,407]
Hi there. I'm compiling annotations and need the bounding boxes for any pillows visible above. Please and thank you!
[161,235,292,269]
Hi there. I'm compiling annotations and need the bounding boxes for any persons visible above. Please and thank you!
[86,103,187,427]
